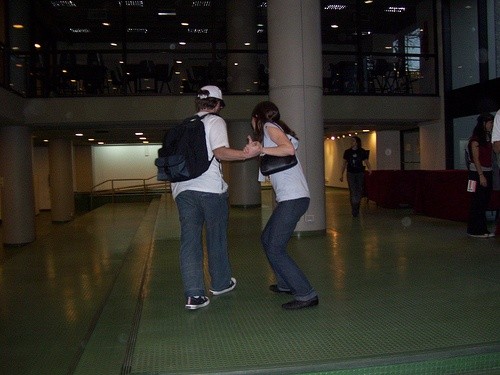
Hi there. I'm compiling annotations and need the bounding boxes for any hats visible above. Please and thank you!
[198,85,225,106]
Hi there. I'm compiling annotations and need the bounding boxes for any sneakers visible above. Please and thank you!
[185,295,209,308]
[209,276,235,294]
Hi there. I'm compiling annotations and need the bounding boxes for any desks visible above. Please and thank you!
[367,169,500,230]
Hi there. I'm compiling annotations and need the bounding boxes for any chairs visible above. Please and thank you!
[27,58,222,97]
[327,59,412,97]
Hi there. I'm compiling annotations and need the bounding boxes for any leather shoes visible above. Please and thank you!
[270,284,292,292]
[282,295,319,309]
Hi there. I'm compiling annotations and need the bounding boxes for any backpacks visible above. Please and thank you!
[158,111,223,181]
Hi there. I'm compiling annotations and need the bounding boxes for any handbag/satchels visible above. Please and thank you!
[259,122,298,175]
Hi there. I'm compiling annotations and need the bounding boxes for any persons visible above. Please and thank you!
[340,136,372,217]
[170,85,262,309]
[467,110,500,238]
[243,102,318,310]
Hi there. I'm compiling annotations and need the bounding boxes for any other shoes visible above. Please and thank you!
[468,230,495,236]
